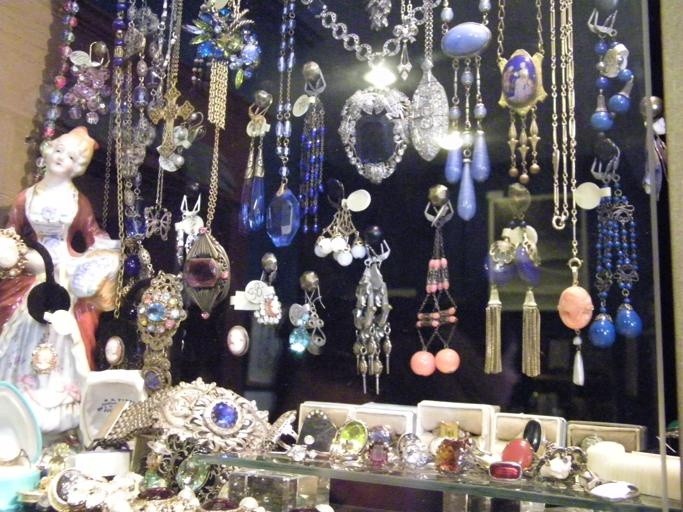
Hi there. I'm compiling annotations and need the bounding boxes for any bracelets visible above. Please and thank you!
[84,373,270,461]
[0,223,26,281]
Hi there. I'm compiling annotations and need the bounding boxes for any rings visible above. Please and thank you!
[270,409,490,476]
[487,420,641,499]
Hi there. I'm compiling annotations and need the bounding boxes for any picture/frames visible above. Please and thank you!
[490,193,589,316]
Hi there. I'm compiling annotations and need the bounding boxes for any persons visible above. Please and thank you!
[0,123,129,439]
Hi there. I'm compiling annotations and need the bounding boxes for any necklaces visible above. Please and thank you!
[29,0,641,387]
